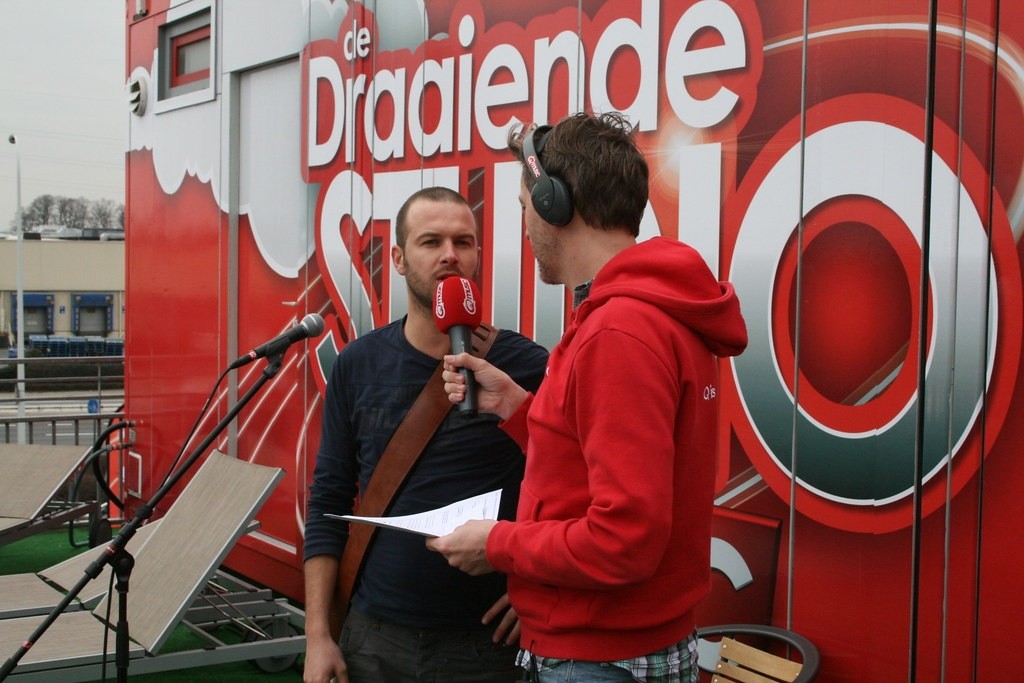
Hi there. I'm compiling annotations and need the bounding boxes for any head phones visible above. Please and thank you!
[521,124,573,227]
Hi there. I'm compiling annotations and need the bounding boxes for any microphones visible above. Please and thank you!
[230,313,326,369]
[433,275,483,419]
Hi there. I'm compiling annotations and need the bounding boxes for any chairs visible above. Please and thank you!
[696,624,821,683]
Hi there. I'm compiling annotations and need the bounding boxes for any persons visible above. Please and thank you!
[424,111,749,683]
[303,185,551,683]
